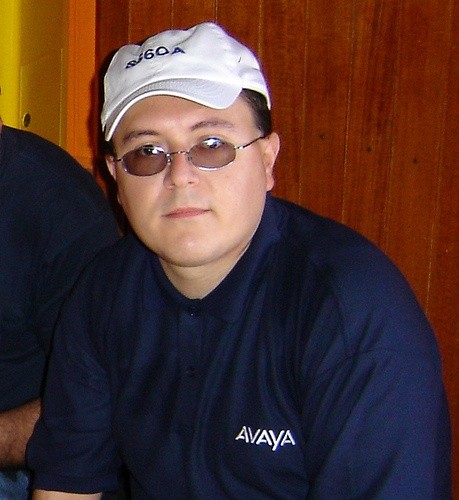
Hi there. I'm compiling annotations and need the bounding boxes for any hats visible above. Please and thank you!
[101,22,271,141]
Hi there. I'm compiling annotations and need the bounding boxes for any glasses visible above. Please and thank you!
[113,134,267,176]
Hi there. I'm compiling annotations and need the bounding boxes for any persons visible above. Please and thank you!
[29,22,451,499]
[0,106,124,500]
